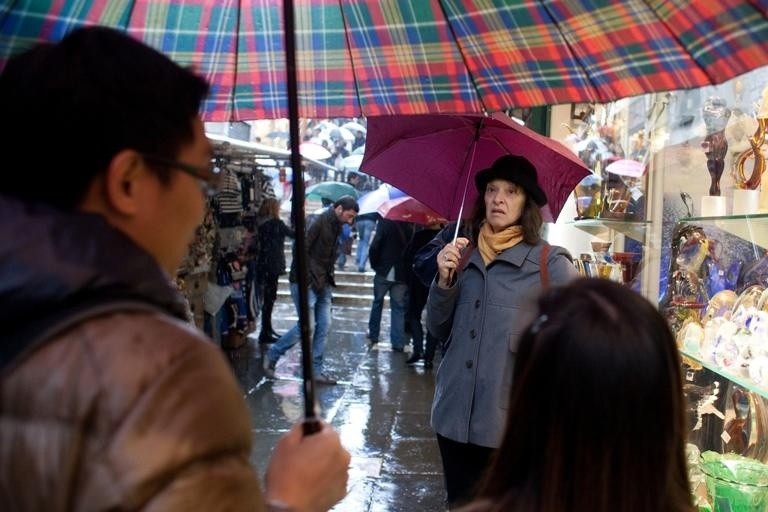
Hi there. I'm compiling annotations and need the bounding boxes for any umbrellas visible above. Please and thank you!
[357,106,595,261]
[0,1,767,440]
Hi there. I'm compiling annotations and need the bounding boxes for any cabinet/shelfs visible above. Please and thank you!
[556,67,768,512]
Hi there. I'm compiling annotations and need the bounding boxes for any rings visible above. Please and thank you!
[444,254,449,261]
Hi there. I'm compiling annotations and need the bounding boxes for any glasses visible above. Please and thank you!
[141,155,223,198]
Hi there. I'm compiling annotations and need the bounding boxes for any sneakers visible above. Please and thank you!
[337,257,368,273]
[258,334,436,387]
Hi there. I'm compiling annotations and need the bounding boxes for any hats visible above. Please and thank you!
[474,157,548,209]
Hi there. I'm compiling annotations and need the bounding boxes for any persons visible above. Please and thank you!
[253,118,438,386]
[0,25,349,510]
[423,154,576,512]
[452,277,701,510]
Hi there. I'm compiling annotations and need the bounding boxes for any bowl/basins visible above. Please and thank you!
[591,241,612,253]
[698,460,768,511]
